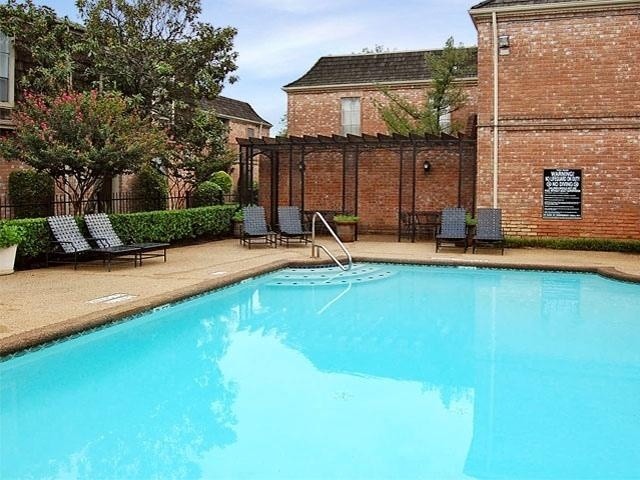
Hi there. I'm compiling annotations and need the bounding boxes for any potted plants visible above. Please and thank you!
[334,214,361,242]
[465,213,477,246]
[0,220,27,276]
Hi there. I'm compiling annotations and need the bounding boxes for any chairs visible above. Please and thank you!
[474,208,505,255]
[47,214,170,270]
[400,211,441,240]
[276,205,312,248]
[240,206,277,250]
[435,208,468,253]
[301,210,353,234]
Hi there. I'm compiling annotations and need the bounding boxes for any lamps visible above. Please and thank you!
[299,161,306,172]
[423,161,431,172]
